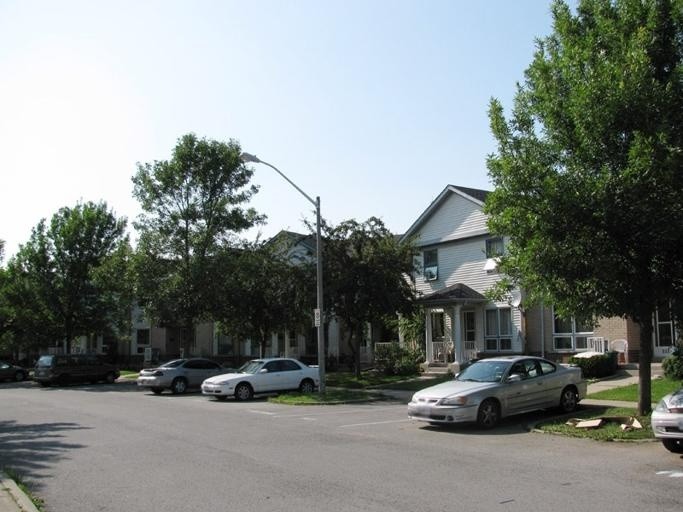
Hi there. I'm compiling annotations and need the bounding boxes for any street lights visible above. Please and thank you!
[240,151,328,395]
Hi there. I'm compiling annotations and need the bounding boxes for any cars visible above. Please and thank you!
[406,356,588,430]
[650,381,683,453]
[135,358,234,395]
[202,356,319,402]
[0,361,28,382]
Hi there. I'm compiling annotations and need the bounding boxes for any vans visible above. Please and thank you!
[31,352,122,386]
